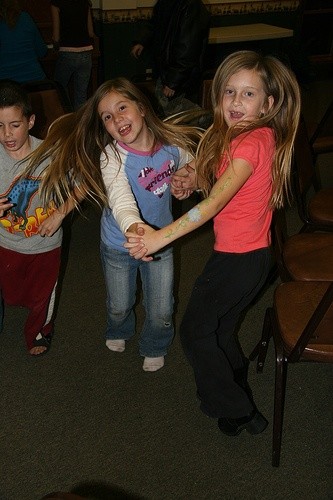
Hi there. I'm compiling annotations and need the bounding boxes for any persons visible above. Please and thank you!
[50,0,96,112]
[12,79,208,371]
[0,0,52,93]
[130,0,212,126]
[1,80,88,357]
[170,50,301,436]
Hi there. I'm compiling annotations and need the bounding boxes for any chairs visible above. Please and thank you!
[26,81,71,143]
[258,101,333,467]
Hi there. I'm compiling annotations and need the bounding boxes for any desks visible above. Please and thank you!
[207,22,293,44]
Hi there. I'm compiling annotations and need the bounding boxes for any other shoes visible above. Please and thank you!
[105,339,126,352]
[142,356,164,372]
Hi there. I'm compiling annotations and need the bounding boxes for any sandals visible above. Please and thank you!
[25,330,54,356]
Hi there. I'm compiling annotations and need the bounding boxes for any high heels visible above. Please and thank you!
[201,383,268,437]
